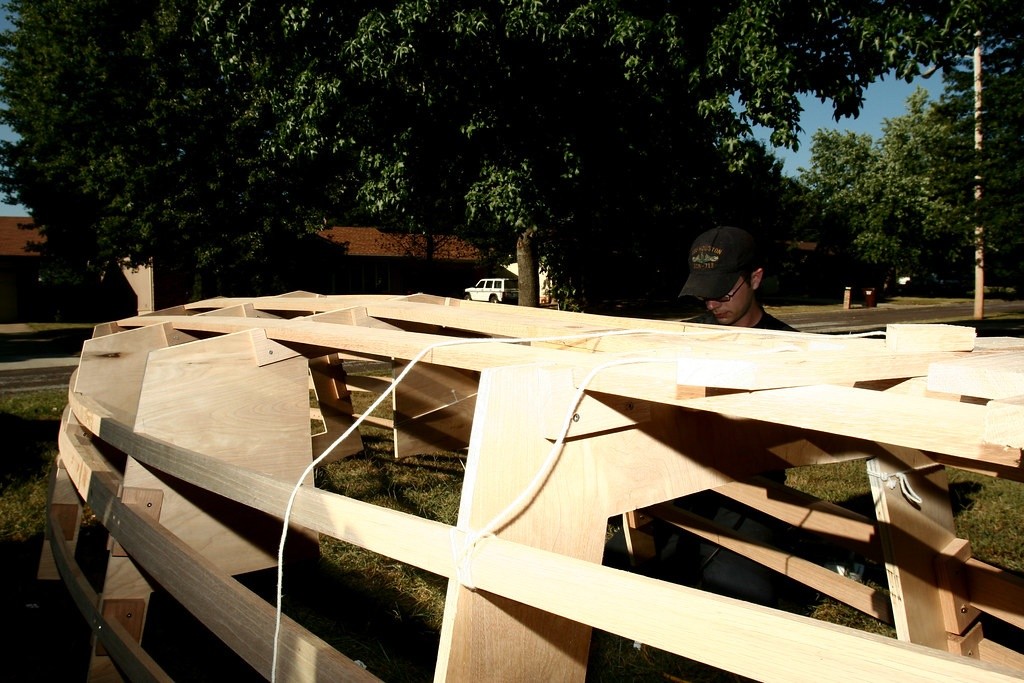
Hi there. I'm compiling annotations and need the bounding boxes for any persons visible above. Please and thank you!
[651,226,786,607]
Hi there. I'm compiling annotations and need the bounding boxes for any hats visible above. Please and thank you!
[678,226,751,299]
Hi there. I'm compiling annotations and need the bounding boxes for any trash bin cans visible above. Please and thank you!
[863,287,876,308]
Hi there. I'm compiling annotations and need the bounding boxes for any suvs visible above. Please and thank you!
[463,278,518,303]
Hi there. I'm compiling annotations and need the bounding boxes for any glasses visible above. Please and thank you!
[695,278,747,303]
